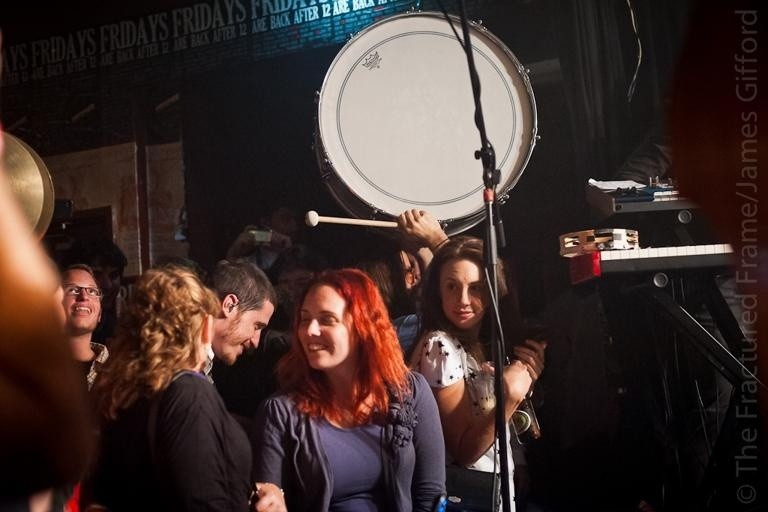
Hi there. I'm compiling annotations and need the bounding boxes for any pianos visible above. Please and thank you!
[569,242,737,286]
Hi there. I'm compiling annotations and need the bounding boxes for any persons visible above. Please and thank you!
[0,119,102,475]
[28,259,126,511]
[244,268,451,510]
[199,251,279,387]
[406,233,552,511]
[95,262,258,510]
[594,142,736,510]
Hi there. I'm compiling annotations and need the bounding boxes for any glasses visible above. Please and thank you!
[60,284,105,298]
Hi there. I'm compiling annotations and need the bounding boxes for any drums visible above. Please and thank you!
[314,10,538,236]
